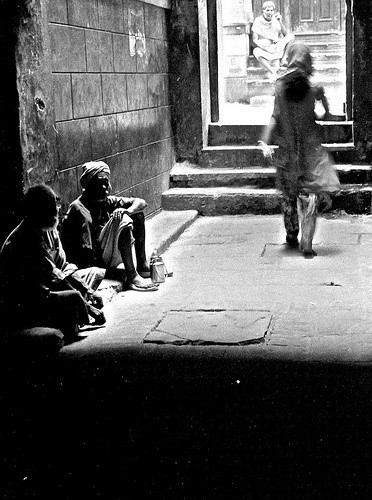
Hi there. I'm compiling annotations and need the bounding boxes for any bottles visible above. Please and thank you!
[150,257,168,283]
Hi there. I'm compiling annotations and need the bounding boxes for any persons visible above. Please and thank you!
[250,1,295,82]
[0,184,105,343]
[61,160,174,289]
[258,41,342,257]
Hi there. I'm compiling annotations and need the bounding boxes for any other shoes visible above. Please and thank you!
[302,247,313,258]
[286,235,299,248]
